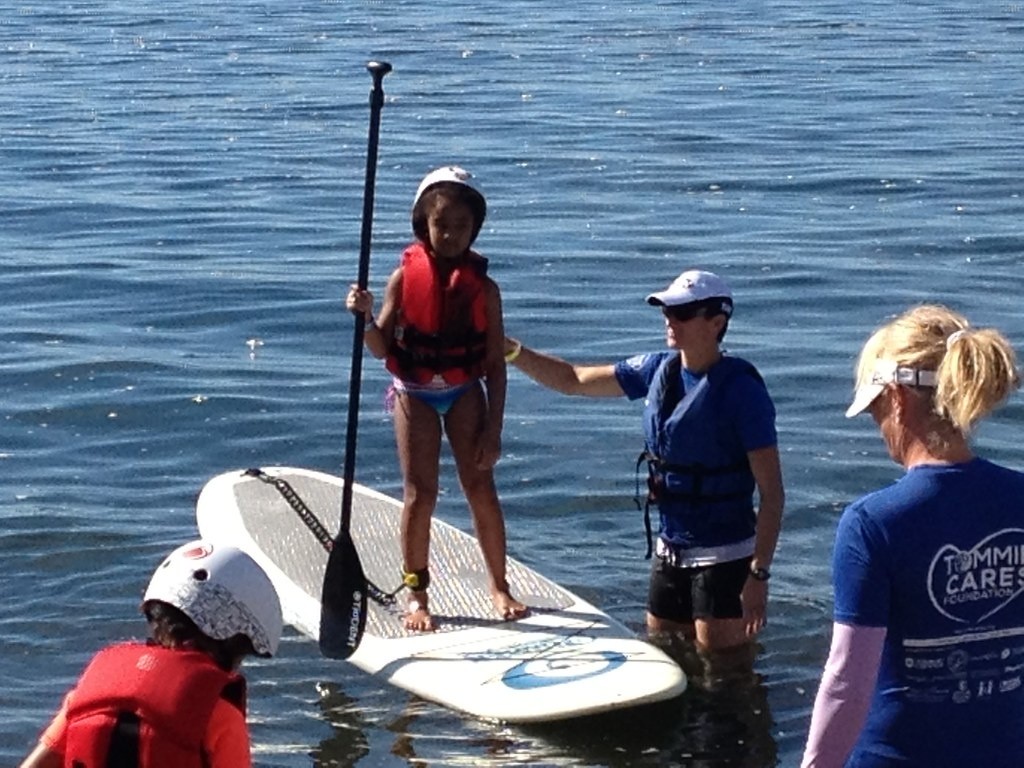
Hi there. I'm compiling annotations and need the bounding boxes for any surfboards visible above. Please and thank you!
[195,466,688,724]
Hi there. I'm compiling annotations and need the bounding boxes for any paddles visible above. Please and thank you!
[309,59,394,663]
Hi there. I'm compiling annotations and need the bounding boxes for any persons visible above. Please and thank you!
[502,269,785,653]
[796,304,1024,768]
[14,541,283,768]
[345,165,531,632]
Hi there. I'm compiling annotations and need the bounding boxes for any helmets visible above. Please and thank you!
[140,539,283,658]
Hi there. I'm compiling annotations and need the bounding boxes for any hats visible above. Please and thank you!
[411,165,487,247]
[645,269,733,307]
[845,330,936,418]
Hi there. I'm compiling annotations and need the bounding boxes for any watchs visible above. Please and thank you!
[744,565,773,583]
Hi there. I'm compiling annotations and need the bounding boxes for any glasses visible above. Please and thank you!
[662,304,706,322]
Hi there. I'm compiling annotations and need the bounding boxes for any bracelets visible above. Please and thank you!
[503,340,521,364]
